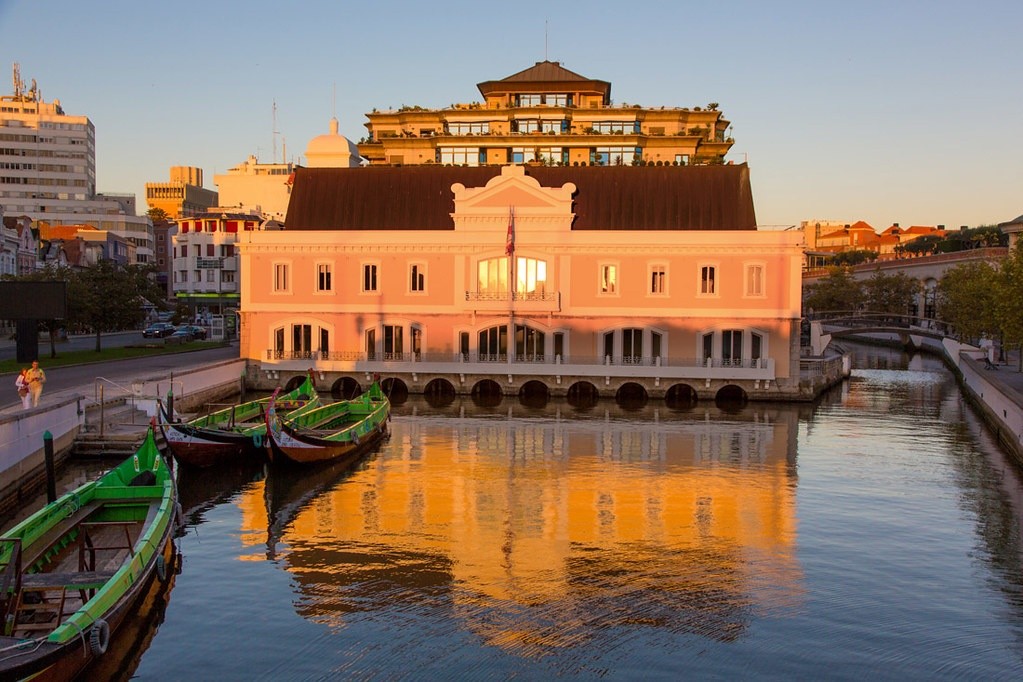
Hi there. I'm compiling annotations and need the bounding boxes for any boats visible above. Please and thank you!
[70,532,183,682]
[155,366,319,470]
[0,426,180,682]
[265,373,392,465]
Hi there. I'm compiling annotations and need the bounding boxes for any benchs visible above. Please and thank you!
[306,410,373,435]
[218,405,301,430]
[984,357,999,370]
[0,497,162,594]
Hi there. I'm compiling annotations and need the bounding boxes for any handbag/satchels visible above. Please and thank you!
[19,389,27,397]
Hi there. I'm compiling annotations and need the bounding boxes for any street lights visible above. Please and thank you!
[219,252,238,313]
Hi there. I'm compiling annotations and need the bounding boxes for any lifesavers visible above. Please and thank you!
[157,554,166,582]
[351,429,359,445]
[158,599,167,625]
[376,424,383,434]
[388,413,391,422]
[90,618,111,655]
[151,416,157,437]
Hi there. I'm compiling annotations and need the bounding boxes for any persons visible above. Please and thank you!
[25,360,47,407]
[15,368,32,409]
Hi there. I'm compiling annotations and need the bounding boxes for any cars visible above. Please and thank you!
[141,322,176,339]
[172,325,207,342]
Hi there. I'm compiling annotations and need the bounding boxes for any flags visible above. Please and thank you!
[505,215,513,256]
